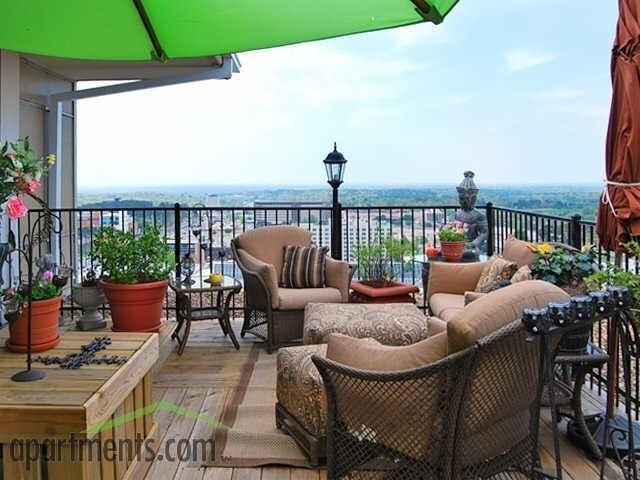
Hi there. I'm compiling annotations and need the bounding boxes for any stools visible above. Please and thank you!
[273,338,386,465]
[302,302,428,345]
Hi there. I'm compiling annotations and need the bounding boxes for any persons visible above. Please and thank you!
[436,171,490,262]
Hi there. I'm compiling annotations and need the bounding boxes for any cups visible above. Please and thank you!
[209,274,223,283]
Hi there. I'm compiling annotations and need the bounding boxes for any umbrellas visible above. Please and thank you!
[0,0,459,65]
[594,0,640,417]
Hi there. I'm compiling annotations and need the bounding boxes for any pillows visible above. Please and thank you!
[279,245,329,288]
[511,264,532,283]
[474,255,517,293]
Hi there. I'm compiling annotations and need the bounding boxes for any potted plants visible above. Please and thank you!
[439,232,465,261]
[88,223,174,333]
[72,280,109,330]
[349,231,427,302]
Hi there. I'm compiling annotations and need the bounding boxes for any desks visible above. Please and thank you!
[0,332,159,480]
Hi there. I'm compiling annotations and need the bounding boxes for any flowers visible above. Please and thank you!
[525,236,597,292]
[1,135,57,221]
[1,271,61,300]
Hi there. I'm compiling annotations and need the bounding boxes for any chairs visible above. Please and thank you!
[311,281,571,480]
[230,226,358,354]
[425,235,587,322]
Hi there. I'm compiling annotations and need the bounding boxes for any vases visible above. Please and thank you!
[4,292,62,352]
[559,327,592,351]
